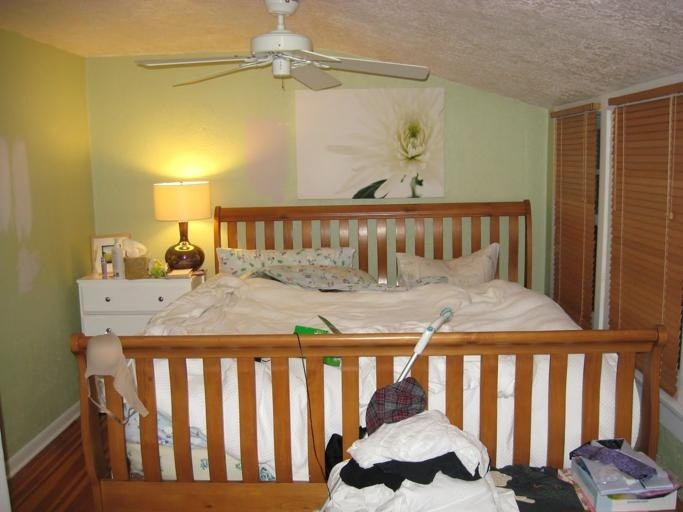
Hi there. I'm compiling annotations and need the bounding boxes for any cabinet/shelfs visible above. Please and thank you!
[77,268,206,414]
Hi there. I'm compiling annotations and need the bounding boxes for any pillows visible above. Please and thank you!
[216,243,500,291]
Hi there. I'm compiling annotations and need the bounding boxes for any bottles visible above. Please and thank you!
[111,239,125,280]
[101,257,108,279]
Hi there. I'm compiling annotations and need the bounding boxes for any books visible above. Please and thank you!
[166,269,193,278]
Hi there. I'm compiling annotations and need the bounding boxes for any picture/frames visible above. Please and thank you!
[90,232,131,276]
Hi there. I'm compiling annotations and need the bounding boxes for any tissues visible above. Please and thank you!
[124,239,147,279]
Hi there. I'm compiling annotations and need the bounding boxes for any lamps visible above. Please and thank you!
[152,181,212,271]
[271,56,291,90]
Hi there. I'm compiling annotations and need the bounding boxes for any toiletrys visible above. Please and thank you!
[112,238,124,278]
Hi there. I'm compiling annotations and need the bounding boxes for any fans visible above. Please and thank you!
[134,0,429,91]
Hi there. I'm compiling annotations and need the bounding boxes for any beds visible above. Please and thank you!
[69,200,669,512]
